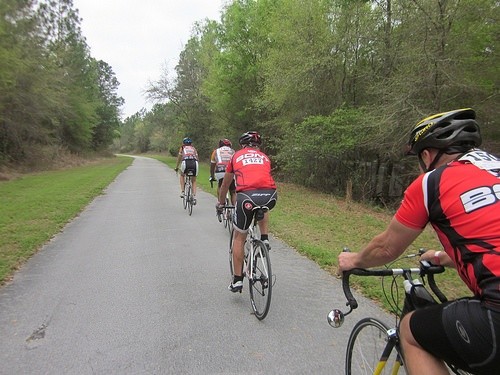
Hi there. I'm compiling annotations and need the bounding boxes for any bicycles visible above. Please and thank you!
[174,167,197,216]
[208,177,237,235]
[327,247,481,375]
[215,201,277,321]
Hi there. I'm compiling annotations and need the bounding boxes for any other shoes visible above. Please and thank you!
[226,211,235,218]
[254,240,270,258]
[193,196,196,205]
[181,192,184,198]
[227,280,243,290]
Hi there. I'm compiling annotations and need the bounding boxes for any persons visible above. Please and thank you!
[209,139,237,212]
[216,132,278,290]
[335,107,500,374]
[175,137,198,201]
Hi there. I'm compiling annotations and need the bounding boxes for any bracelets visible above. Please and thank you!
[434,251,442,265]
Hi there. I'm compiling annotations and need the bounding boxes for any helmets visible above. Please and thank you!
[183,136,192,144]
[404,107,477,157]
[219,139,232,148]
[239,131,262,148]
